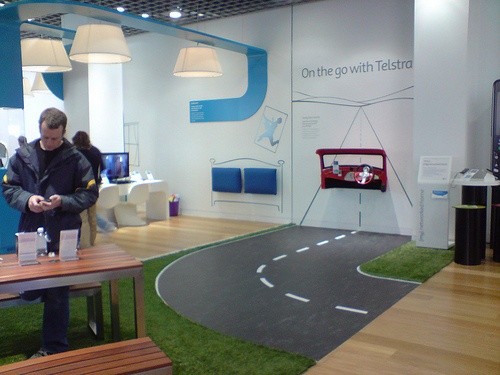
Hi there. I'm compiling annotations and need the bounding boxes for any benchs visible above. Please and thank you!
[0,282,106,338]
[0,335,174,375]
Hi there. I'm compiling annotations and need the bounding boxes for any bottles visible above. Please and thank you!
[37,227,48,256]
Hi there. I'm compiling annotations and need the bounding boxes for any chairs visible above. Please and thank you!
[97,185,119,211]
[113,182,150,226]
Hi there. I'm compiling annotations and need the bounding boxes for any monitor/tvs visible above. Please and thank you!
[100,152,129,179]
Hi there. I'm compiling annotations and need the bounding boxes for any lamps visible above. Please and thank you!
[172,5,223,77]
[69,19,132,63]
[21,17,73,73]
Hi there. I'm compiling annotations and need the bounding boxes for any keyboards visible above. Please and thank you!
[109,180,135,184]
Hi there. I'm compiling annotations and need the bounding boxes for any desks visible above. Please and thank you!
[453,178,500,264]
[0,245,145,337]
[97,179,172,224]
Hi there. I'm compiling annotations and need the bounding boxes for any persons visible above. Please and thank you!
[17,134,28,148]
[69,130,105,248]
[0,108,100,362]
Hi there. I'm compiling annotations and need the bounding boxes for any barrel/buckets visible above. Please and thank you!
[169,201,180,216]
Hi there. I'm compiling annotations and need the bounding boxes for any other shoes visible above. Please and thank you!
[28,348,52,359]
